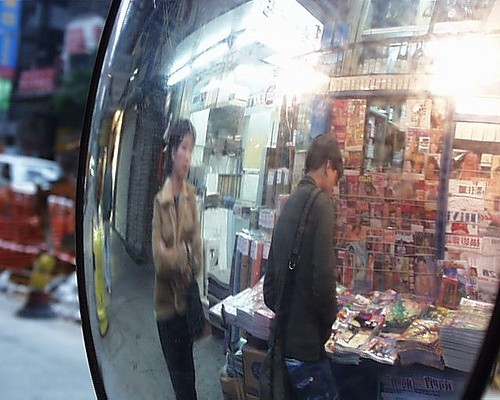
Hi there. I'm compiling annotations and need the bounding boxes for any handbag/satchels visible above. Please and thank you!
[259,351,289,400]
[185,275,211,342]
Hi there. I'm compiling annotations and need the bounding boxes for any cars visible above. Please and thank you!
[0,153,75,218]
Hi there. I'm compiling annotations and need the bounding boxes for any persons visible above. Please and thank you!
[150,119,213,400]
[262,134,346,400]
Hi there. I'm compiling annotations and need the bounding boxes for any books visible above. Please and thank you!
[201,93,500,378]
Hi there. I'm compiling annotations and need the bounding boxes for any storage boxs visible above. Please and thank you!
[218,373,244,400]
[241,339,305,400]
[381,364,469,400]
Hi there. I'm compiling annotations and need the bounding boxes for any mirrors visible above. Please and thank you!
[74,0,500,400]
